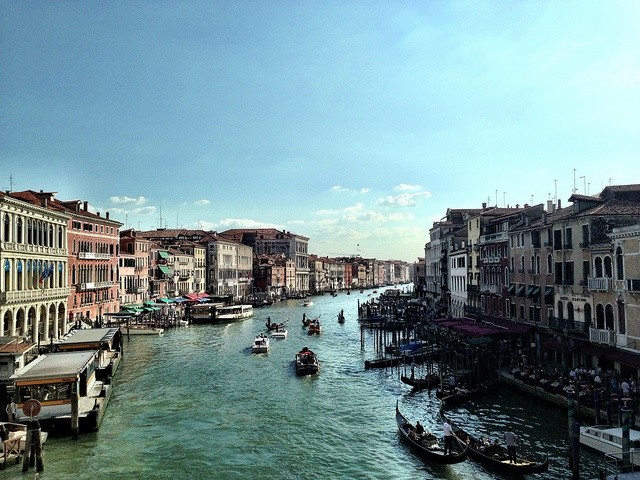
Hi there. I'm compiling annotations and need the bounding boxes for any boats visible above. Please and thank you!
[215,305,253,322]
[235,289,273,308]
[439,406,548,474]
[190,302,224,322]
[271,329,287,339]
[365,337,439,367]
[252,333,269,352]
[266,316,289,330]
[579,425,640,466]
[285,289,312,299]
[396,400,470,463]
[146,298,188,327]
[308,320,321,335]
[401,371,446,387]
[103,313,164,335]
[296,347,318,376]
[356,293,410,328]
[0,422,48,451]
[302,314,321,326]
[436,384,487,401]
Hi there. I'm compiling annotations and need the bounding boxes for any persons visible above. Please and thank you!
[594,375,603,389]
[340,309,344,320]
[483,438,504,457]
[410,360,417,379]
[614,373,639,399]
[6,396,17,422]
[443,420,454,455]
[448,374,456,393]
[504,427,517,464]
[566,363,614,385]
[415,421,424,436]
[302,312,306,324]
[266,316,271,326]
